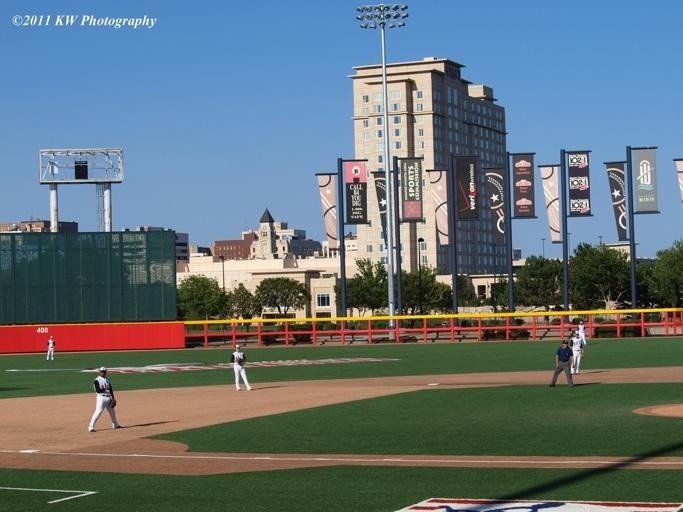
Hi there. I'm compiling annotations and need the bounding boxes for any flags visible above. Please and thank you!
[629,147,657,211]
[565,152,592,216]
[452,155,480,220]
[425,169,450,247]
[483,168,506,245]
[603,162,628,240]
[371,172,393,248]
[342,159,369,224]
[509,154,536,217]
[314,173,339,250]
[673,160,683,204]
[398,158,424,221]
[538,166,561,242]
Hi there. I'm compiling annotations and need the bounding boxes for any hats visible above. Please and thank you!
[574,331,579,335]
[234,344,241,348]
[49,335,54,339]
[99,366,107,371]
[579,320,583,324]
[562,339,569,344]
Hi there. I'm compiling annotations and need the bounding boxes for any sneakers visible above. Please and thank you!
[236,388,242,393]
[570,372,580,377]
[568,383,576,387]
[88,425,98,432]
[548,383,556,388]
[111,423,124,429]
[247,388,256,391]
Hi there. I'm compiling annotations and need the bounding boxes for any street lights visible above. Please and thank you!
[540,237,546,257]
[567,232,571,257]
[416,236,425,316]
[218,254,225,292]
[598,235,603,244]
[356,1,409,340]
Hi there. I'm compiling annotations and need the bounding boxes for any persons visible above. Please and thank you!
[230,344,252,392]
[86,366,124,432]
[569,330,584,375]
[44,334,56,362]
[547,339,577,387]
[575,321,586,346]
